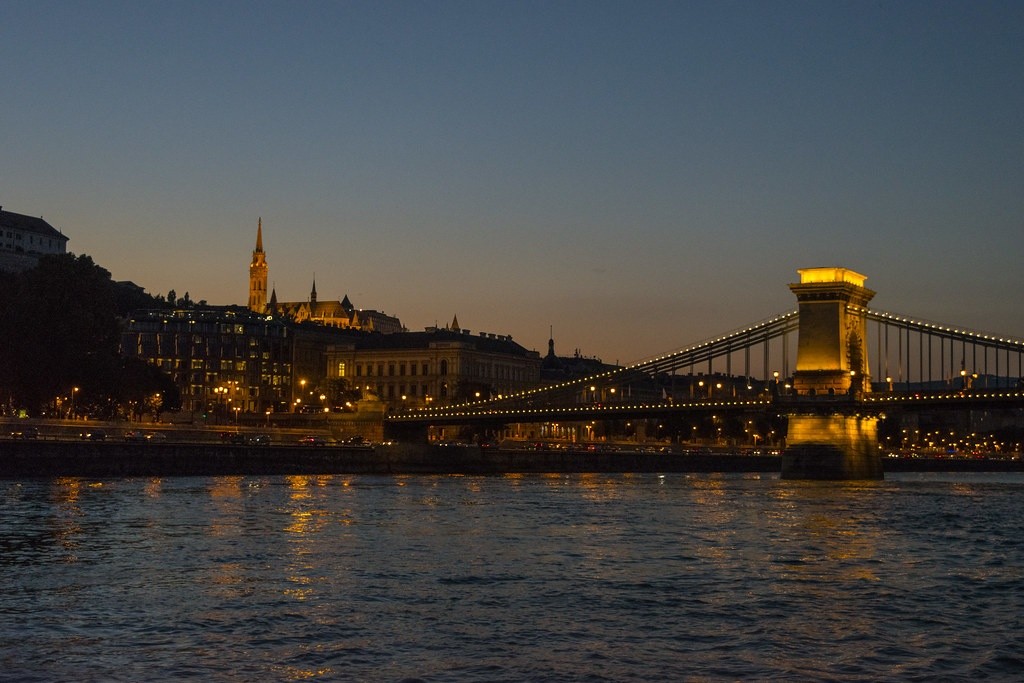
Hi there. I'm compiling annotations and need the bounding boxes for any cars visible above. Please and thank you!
[221,432,245,444]
[741,449,754,456]
[143,432,166,443]
[11,427,40,440]
[640,447,655,453]
[250,435,271,444]
[124,432,144,441]
[298,436,325,446]
[433,437,499,450]
[81,429,107,441]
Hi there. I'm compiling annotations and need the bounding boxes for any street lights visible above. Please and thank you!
[219,387,227,425]
[233,407,241,426]
[214,388,218,425]
[72,386,78,404]
[586,426,591,440]
